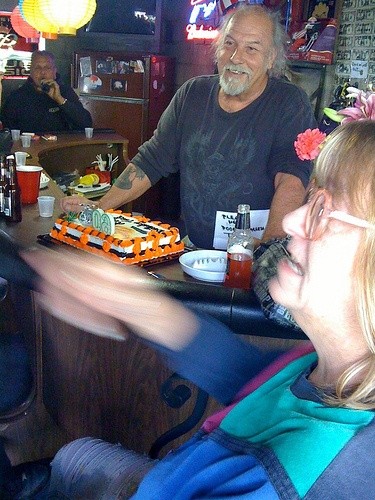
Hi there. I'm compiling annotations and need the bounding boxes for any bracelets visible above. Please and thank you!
[63,98,67,104]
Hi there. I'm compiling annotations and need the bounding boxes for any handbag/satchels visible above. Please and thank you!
[248,233,303,333]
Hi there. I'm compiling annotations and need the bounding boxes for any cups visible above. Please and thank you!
[85,128,93,138]
[95,169,110,185]
[11,130,20,140]
[14,151,27,165]
[21,135,31,147]
[37,196,55,218]
[16,165,43,204]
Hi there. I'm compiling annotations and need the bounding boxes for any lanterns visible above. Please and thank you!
[10,0,97,44]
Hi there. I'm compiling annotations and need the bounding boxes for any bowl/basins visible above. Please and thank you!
[22,133,35,137]
[40,177,50,189]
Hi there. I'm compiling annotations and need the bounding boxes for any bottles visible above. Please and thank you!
[0,155,8,213]
[4,158,22,222]
[42,78,50,94]
[223,203,255,290]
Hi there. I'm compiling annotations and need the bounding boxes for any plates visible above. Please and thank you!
[179,249,226,280]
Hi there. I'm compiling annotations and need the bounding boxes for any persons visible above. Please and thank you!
[61,6,317,251]
[21,118,375,500]
[0,51,92,133]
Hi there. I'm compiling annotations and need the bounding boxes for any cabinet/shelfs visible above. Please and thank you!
[78,50,175,219]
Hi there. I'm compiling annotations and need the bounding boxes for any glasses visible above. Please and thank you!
[303,177,375,242]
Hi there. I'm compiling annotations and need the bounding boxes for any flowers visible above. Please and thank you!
[294,128,325,161]
[323,86,375,125]
